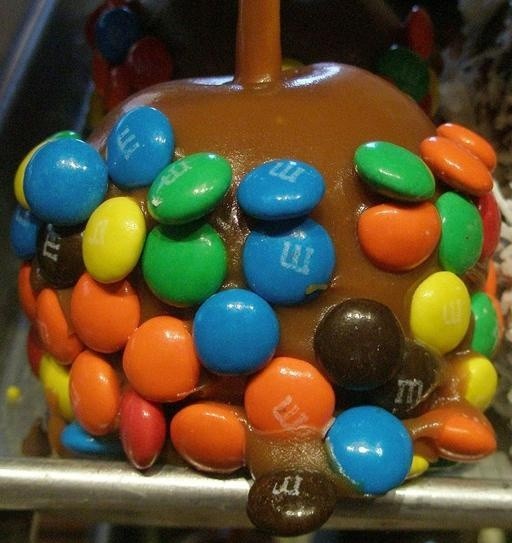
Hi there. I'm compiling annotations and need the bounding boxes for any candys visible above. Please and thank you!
[10,0,502,537]
